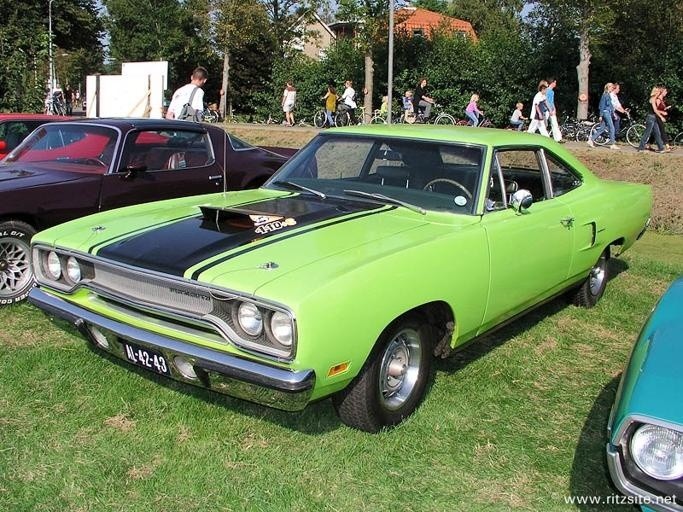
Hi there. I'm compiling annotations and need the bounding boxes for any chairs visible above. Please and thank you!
[367,165,410,186]
[99,143,128,173]
[18,134,37,150]
[422,163,491,198]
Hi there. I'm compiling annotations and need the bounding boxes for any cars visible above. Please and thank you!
[26,123,654,435]
[82,97,86,110]
[605,273,682,511]
[0,112,189,169]
[0,117,318,306]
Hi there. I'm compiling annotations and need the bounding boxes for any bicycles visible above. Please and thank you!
[162,95,683,151]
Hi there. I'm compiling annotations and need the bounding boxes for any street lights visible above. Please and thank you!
[52,54,68,89]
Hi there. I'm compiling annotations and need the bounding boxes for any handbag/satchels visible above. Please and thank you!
[179,103,204,122]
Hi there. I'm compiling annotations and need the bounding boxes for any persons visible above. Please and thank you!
[280,77,673,154]
[44,82,81,117]
[165,66,209,168]
